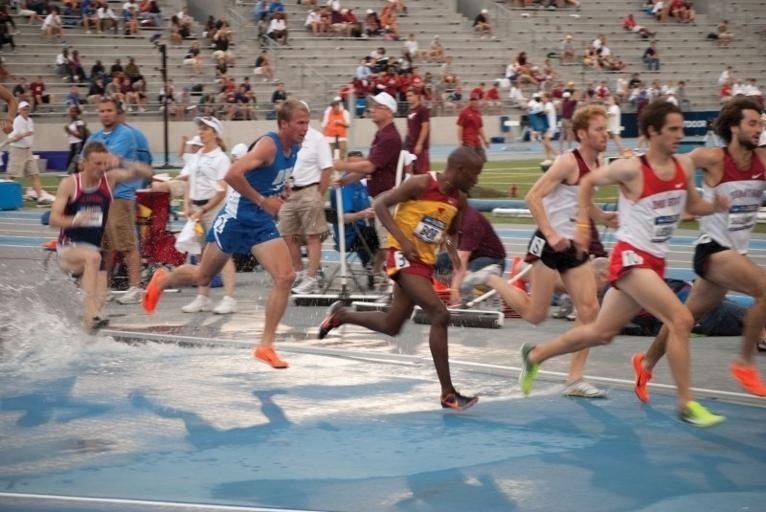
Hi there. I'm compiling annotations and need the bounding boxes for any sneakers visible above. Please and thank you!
[518,341,542,398]
[254,343,289,369]
[210,295,239,315]
[669,395,729,430]
[440,388,478,412]
[552,299,572,319]
[566,306,578,321]
[141,267,166,315]
[115,287,143,306]
[459,262,505,297]
[724,360,764,400]
[630,351,655,405]
[290,276,322,297]
[558,377,607,398]
[317,299,348,340]
[34,196,56,205]
[181,293,215,313]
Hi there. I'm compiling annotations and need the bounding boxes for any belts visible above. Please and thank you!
[287,181,322,193]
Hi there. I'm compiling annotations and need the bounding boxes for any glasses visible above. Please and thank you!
[97,94,116,105]
[467,96,479,102]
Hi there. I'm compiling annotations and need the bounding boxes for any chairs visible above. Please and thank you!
[325,209,393,297]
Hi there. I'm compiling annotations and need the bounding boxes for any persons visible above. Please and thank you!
[253,49,278,82]
[158,70,258,126]
[470,82,486,99]
[582,35,626,70]
[12,77,45,113]
[41,5,66,43]
[643,39,660,71]
[144,99,310,368]
[530,91,558,170]
[402,34,422,57]
[558,92,579,152]
[518,101,726,430]
[0,21,17,54]
[604,96,626,153]
[42,92,151,250]
[124,58,139,74]
[472,8,493,35]
[299,0,408,38]
[487,82,504,115]
[4,100,58,204]
[64,104,89,164]
[281,100,333,296]
[427,33,447,63]
[457,93,490,151]
[166,10,194,44]
[91,59,104,78]
[52,46,86,81]
[111,58,123,72]
[559,35,578,65]
[10,0,43,25]
[402,86,432,175]
[182,41,201,64]
[633,95,766,403]
[229,142,249,162]
[182,114,239,314]
[254,0,290,46]
[321,97,352,160]
[640,2,697,23]
[459,105,620,399]
[0,81,18,134]
[82,99,154,304]
[203,15,234,50]
[709,19,735,46]
[329,150,388,290]
[502,51,560,90]
[506,0,580,9]
[528,74,688,122]
[317,144,487,411]
[64,71,148,115]
[335,91,402,275]
[624,12,657,38]
[440,55,462,86]
[80,0,161,35]
[720,65,763,96]
[177,135,205,161]
[342,48,435,118]
[49,141,135,329]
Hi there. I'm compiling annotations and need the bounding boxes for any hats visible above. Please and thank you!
[194,115,224,140]
[367,89,400,117]
[346,149,363,160]
[563,92,571,98]
[17,101,31,111]
